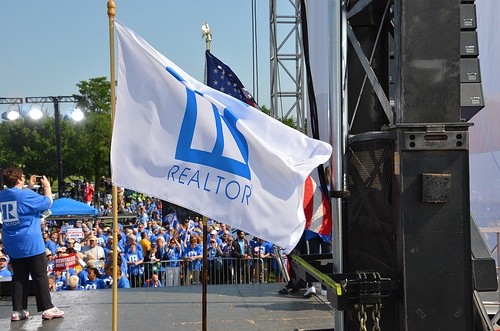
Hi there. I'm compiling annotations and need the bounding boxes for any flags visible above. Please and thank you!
[110,19,334,249]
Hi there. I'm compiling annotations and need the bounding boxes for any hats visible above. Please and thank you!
[210,239,216,242]
[210,229,217,234]
[0,255,8,260]
[90,236,97,240]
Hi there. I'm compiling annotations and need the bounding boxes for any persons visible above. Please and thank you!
[82,236,105,268]
[84,268,107,289]
[36,176,289,291]
[125,244,143,288]
[0,256,11,277]
[0,166,64,321]
[302,164,332,298]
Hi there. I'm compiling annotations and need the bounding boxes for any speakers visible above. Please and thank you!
[338,129,474,331]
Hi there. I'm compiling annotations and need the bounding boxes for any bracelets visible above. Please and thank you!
[44,187,51,189]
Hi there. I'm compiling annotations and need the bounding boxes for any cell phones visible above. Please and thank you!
[35,176,43,183]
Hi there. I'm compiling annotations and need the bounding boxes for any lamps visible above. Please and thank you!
[2,108,85,123]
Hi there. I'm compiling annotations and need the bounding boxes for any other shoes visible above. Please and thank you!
[42,307,64,319]
[11,309,29,321]
[304,286,316,297]
[279,287,298,295]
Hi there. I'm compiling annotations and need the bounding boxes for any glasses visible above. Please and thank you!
[46,254,52,257]
